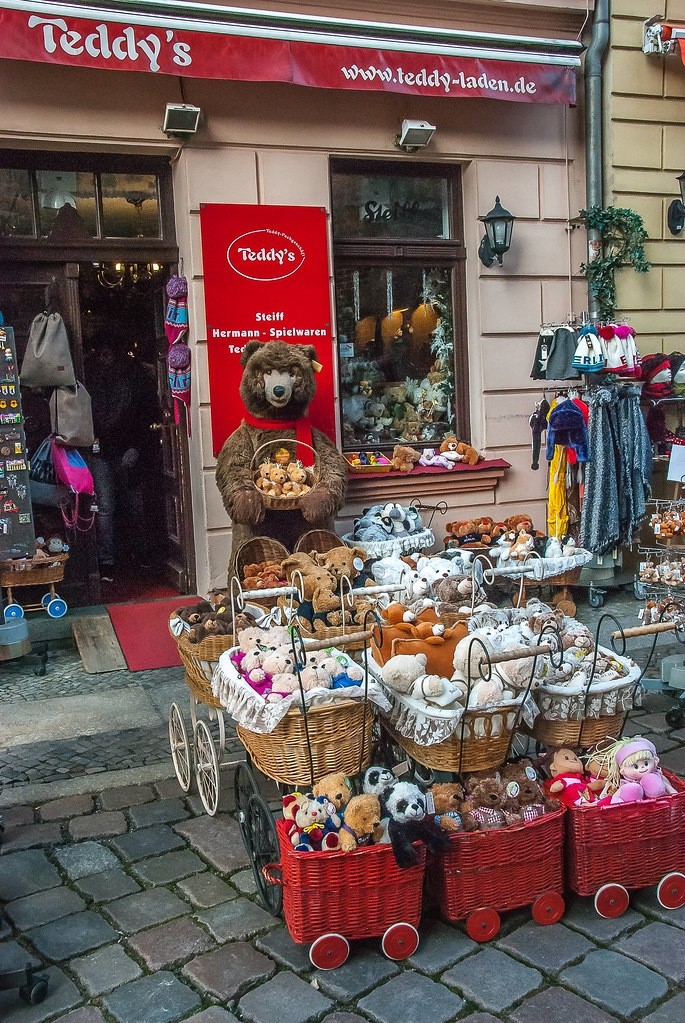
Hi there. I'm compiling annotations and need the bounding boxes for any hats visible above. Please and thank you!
[529,320,685,470]
[163,273,188,348]
[167,345,192,439]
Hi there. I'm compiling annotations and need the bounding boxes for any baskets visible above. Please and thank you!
[250,439,322,510]
[342,451,395,473]
[168,527,642,783]
[0,547,70,620]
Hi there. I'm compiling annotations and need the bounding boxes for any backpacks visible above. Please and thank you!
[18,300,75,387]
[51,435,96,531]
[28,434,70,510]
[48,380,95,447]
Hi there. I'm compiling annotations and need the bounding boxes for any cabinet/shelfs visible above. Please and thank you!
[637,398,685,603]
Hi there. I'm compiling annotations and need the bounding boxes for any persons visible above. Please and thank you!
[83,327,157,582]
[641,352,685,400]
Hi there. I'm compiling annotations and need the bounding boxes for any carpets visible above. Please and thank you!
[106,597,207,672]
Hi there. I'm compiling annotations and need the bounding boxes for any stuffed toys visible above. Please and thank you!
[283,767,452,867]
[217,340,349,586]
[651,510,685,539]
[477,598,626,687]
[370,603,545,709]
[427,758,561,838]
[373,549,498,613]
[642,596,685,634]
[0,534,68,572]
[391,436,479,472]
[444,515,579,561]
[233,626,375,704]
[542,735,677,819]
[353,502,423,542]
[243,560,287,588]
[639,557,685,586]
[281,547,390,638]
[176,589,259,644]
[343,357,456,444]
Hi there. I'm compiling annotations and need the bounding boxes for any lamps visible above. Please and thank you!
[478,195,516,268]
[93,194,165,290]
[668,170,685,235]
[162,101,201,133]
[395,118,437,151]
